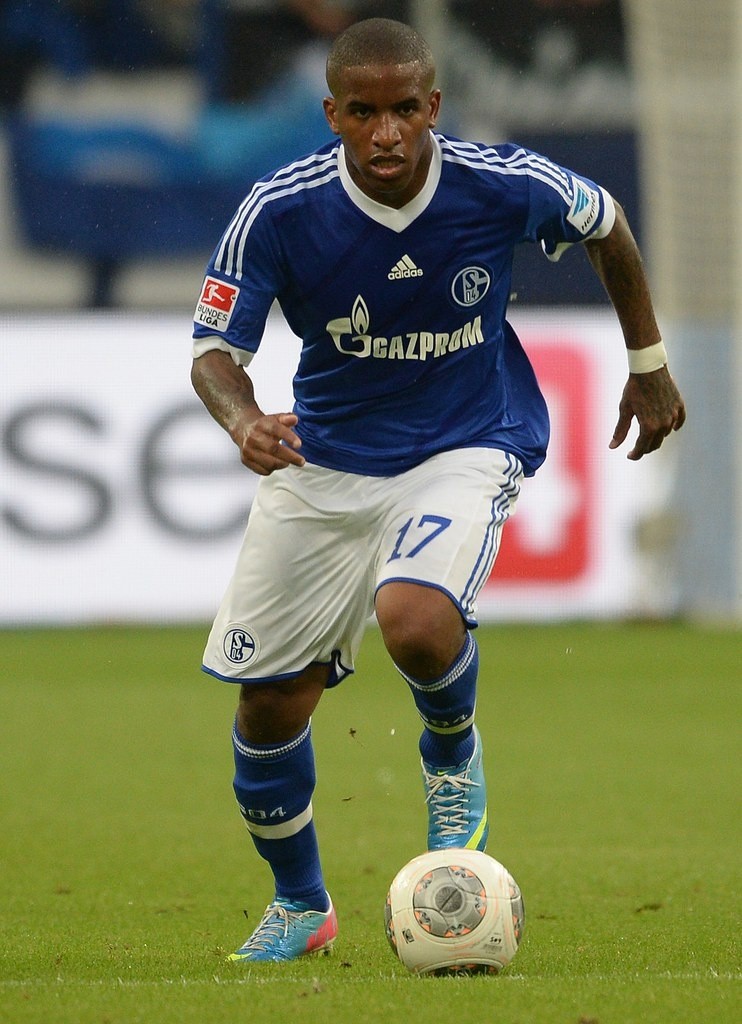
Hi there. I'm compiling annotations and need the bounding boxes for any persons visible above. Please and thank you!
[192,18,685,965]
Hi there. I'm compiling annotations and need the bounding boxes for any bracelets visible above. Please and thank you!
[625,342,668,373]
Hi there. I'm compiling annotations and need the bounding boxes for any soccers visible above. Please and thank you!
[382,848,528,979]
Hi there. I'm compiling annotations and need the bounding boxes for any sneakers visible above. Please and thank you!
[420,722,490,852]
[224,891,340,961]
